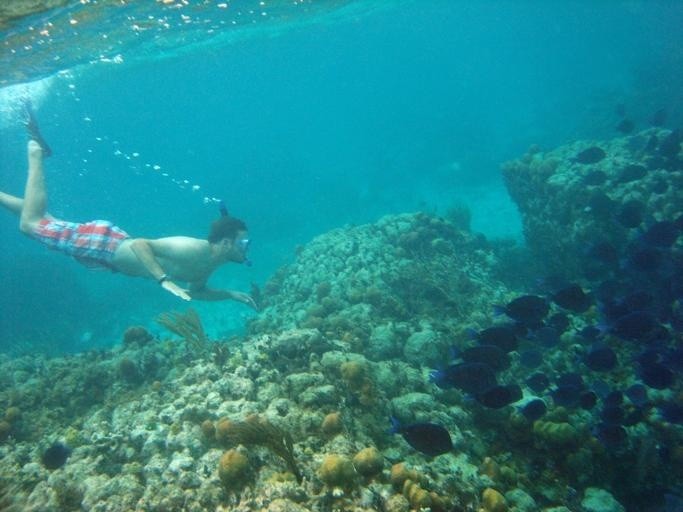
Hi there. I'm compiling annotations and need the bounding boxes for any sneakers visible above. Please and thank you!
[29,130,51,158]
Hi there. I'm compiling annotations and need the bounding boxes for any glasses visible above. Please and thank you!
[233,239,251,253]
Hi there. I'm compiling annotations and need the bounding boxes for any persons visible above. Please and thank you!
[1,96,259,311]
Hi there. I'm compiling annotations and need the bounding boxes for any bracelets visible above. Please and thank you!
[159,275,171,284]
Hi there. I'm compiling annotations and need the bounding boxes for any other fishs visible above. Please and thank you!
[427,272,682,511]
